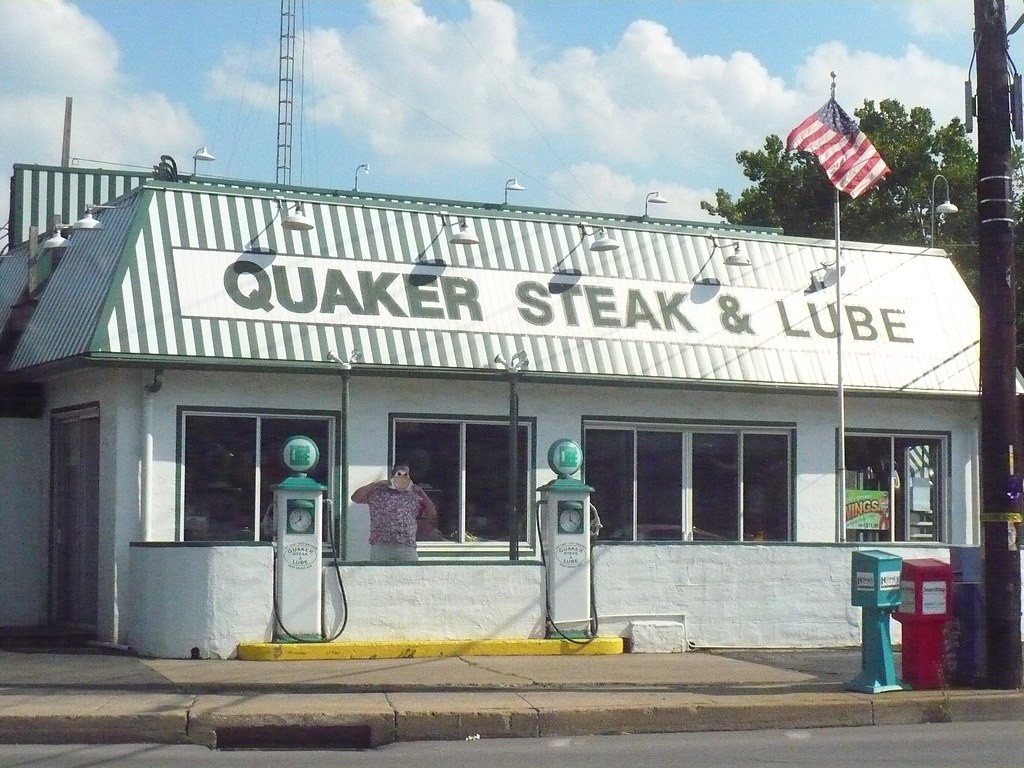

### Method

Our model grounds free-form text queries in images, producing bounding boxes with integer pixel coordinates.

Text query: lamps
[930,174,957,249]
[327,348,364,370]
[643,191,668,217]
[579,222,620,251]
[275,194,314,231]
[440,211,481,244]
[44,203,117,250]
[495,351,529,373]
[502,179,526,205]
[710,233,753,267]
[193,146,216,178]
[820,246,847,287]
[353,163,370,191]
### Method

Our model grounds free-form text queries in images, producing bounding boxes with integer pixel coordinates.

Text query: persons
[351,463,434,561]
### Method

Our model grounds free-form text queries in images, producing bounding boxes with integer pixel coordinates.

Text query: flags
[787,100,893,200]
